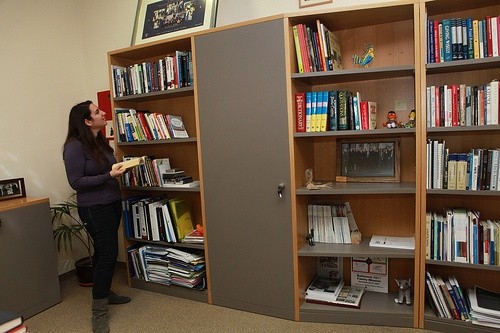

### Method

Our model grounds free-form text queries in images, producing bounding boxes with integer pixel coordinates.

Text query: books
[307,274,365,309]
[427,16,500,63]
[426,80,500,126]
[425,208,500,266]
[114,108,189,142]
[109,48,194,98]
[426,138,500,192]
[425,267,500,329]
[111,156,147,172]
[295,89,376,132]
[293,19,343,73]
[0,310,29,333]
[126,243,206,291]
[369,234,415,250]
[308,199,360,244]
[121,194,205,244]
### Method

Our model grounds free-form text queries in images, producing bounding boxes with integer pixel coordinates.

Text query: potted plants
[50,191,98,287]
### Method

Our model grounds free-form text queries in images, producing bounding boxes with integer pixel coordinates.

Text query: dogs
[394,277,412,304]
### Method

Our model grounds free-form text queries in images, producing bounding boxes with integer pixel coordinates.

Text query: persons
[63,100,132,333]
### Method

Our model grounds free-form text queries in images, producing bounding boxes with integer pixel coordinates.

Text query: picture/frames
[336,136,404,182]
[0,177,26,201]
[130,0,217,46]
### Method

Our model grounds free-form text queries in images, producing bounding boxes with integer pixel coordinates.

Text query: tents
[123,156,199,188]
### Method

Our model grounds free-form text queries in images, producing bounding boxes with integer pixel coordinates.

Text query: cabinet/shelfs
[103,0,500,333]
[0,196,63,320]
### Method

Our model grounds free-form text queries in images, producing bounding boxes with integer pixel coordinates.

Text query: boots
[91,298,110,333]
[109,290,130,304]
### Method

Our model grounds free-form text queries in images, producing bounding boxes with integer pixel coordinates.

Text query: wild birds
[352,41,376,68]
